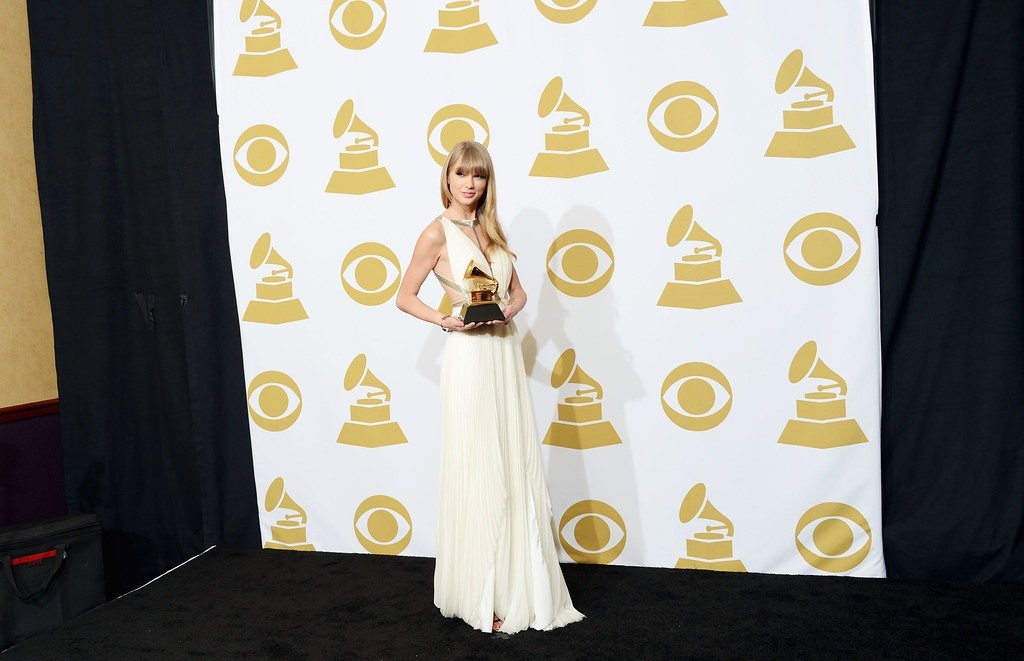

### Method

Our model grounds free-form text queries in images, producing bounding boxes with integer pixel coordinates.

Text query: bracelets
[441,315,454,332]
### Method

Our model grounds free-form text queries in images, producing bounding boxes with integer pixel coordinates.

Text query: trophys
[458,258,506,324]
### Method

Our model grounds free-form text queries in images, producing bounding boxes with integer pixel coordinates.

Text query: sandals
[492,613,504,633]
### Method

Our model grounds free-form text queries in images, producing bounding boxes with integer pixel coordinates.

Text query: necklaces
[450,218,479,226]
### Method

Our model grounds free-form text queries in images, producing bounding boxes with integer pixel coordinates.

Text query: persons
[396,141,561,633]
